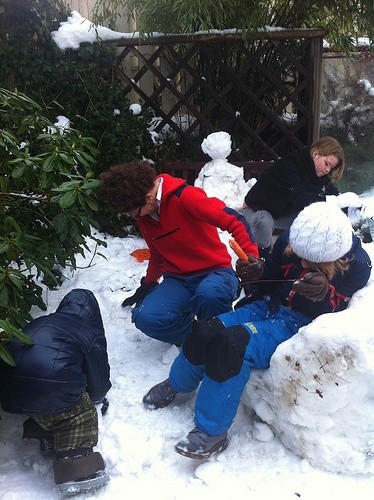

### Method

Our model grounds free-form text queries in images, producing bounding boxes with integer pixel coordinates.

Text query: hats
[289,202,352,263]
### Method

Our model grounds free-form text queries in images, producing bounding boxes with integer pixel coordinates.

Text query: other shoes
[258,246,274,276]
[40,436,54,456]
[52,472,109,498]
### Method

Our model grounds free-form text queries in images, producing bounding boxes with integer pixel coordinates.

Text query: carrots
[229,240,249,262]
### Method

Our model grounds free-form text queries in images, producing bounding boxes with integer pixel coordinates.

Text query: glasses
[124,200,144,220]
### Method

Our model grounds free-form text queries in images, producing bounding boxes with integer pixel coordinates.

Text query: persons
[95,161,261,410]
[143,202,372,461]
[0,288,111,497]
[246,137,345,258]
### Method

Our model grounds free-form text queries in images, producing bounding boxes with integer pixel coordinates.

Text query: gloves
[122,277,160,307]
[236,256,263,282]
[293,272,328,302]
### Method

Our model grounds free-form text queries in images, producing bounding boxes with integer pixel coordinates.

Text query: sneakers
[143,381,178,409]
[176,428,228,460]
